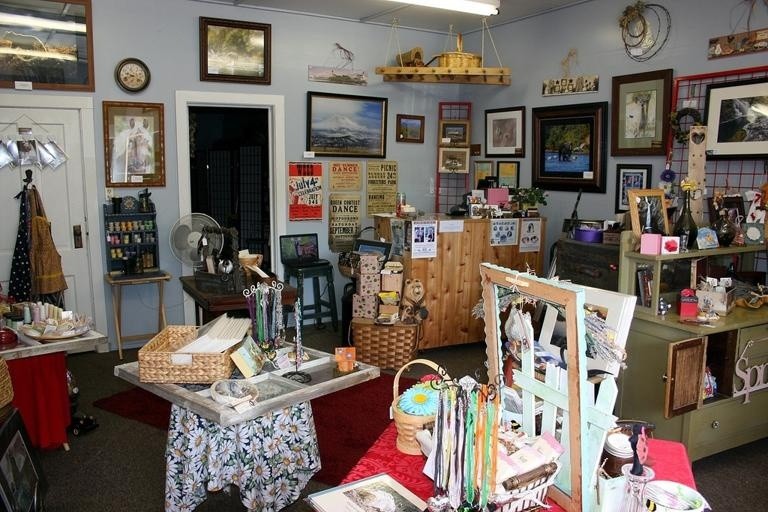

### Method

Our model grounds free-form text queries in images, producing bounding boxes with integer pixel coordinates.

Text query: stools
[283,259,339,332]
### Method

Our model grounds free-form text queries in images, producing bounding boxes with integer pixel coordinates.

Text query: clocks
[114,58,152,94]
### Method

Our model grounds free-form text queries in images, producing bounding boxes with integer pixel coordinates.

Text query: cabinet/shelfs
[103,196,161,274]
[618,230,768,462]
[554,239,691,297]
[374,211,547,349]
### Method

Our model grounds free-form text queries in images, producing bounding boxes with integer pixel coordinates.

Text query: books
[159,312,252,355]
[307,472,428,512]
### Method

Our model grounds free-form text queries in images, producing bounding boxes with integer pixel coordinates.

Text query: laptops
[278,233,329,269]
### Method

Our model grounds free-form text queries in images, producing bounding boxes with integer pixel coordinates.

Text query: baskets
[137,325,239,384]
[0,358,14,408]
[391,358,456,455]
[348,316,421,373]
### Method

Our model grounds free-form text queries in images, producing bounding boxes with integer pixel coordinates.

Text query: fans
[169,213,224,280]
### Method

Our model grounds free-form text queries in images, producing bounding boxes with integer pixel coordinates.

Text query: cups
[680,234,689,256]
[0,313,25,333]
[395,191,406,218]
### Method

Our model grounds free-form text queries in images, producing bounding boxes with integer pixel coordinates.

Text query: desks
[0,311,108,449]
[178,276,296,328]
[115,344,380,512]
[339,416,699,512]
[105,271,171,359]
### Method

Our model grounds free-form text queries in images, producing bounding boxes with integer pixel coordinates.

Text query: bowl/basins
[238,252,263,278]
[575,227,602,243]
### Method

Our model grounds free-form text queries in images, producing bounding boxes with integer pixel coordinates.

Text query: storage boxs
[677,295,699,320]
[602,232,621,245]
[356,255,380,296]
[381,262,404,292]
[378,292,400,318]
[696,285,736,316]
[352,294,378,319]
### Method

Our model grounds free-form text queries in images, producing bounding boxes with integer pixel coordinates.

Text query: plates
[641,481,706,512]
[20,311,93,340]
[604,432,633,460]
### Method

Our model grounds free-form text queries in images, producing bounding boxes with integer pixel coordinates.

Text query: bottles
[710,211,735,247]
[395,192,405,217]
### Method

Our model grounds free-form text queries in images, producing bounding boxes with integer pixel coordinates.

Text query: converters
[317,324,327,329]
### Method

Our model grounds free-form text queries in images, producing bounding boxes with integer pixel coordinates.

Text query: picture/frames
[0,408,41,511]
[439,120,471,148]
[103,101,166,187]
[473,160,494,190]
[199,15,272,85]
[484,105,526,159]
[496,161,520,189]
[637,271,653,307]
[531,101,609,194]
[0,0,95,93]
[708,196,746,226]
[610,68,674,156]
[396,114,425,144]
[615,164,652,214]
[438,148,471,174]
[703,75,768,160]
[306,91,388,158]
[629,188,669,235]
